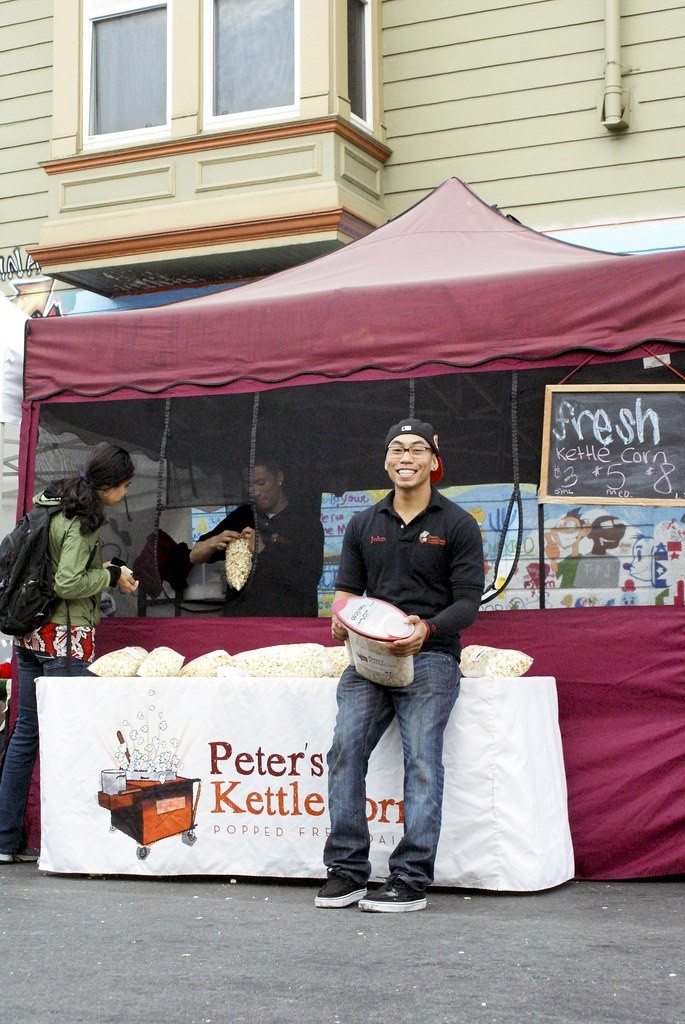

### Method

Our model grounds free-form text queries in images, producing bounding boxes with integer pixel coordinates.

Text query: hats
[384,418,443,486]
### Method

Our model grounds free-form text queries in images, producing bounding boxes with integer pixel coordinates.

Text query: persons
[189,451,325,618]
[0,438,140,860]
[314,419,483,912]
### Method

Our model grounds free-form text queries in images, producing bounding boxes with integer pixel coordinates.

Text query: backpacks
[1,501,100,637]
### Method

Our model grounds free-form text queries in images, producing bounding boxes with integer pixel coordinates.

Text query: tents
[5,175,685,881]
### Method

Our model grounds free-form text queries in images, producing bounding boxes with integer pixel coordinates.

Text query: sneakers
[1,845,39,862]
[315,870,367,908]
[358,875,427,913]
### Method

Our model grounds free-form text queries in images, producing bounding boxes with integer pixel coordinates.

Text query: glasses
[386,446,433,459]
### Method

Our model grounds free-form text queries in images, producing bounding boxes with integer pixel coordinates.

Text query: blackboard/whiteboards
[537,384,685,509]
